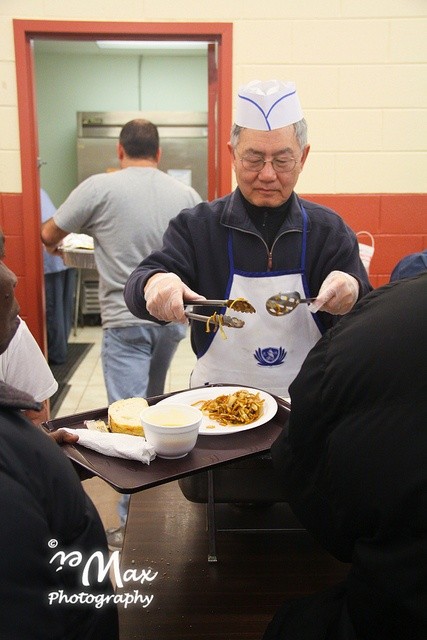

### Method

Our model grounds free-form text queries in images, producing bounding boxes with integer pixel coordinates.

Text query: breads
[107,397,149,436]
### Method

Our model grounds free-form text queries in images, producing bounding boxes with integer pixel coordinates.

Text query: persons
[41,119,204,552]
[0,314,59,427]
[40,186,76,365]
[288,271,427,639]
[123,79,374,406]
[0,230,121,638]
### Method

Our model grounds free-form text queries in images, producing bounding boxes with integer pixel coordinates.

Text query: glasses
[234,147,303,172]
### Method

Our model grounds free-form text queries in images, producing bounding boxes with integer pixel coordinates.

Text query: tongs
[183,297,256,330]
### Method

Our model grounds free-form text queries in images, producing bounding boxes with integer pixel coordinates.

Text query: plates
[158,386,278,437]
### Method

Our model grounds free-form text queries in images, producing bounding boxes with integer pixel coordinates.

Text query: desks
[115,479,353,638]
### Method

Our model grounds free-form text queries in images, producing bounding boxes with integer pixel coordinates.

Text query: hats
[234,80,304,131]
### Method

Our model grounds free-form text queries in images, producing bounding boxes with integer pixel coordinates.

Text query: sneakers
[105,527,125,553]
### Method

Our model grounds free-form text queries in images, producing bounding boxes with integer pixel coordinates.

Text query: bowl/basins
[139,404,203,460]
